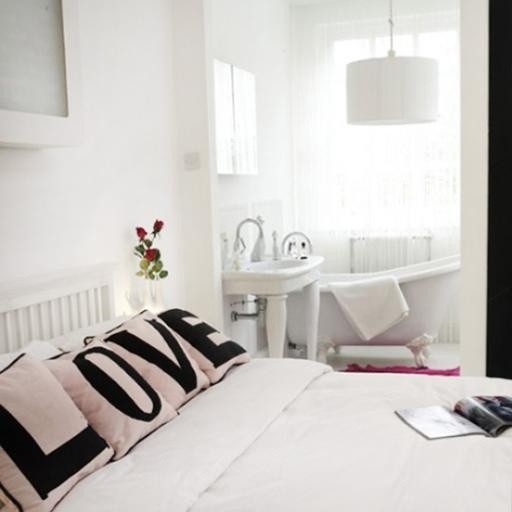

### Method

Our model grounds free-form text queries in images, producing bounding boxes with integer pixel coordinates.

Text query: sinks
[222,254,325,296]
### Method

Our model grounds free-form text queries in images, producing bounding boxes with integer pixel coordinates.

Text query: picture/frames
[0,0,83,149]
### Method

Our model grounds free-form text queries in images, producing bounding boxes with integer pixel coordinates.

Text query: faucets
[281,231,312,256]
[233,218,265,270]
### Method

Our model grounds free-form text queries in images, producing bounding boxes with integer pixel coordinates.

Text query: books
[392,394,512,442]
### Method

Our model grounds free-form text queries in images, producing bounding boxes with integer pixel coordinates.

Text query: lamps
[345,0,441,127]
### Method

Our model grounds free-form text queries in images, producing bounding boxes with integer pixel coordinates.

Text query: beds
[1,263,511,512]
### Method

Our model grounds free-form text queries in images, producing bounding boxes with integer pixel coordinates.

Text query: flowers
[132,220,169,305]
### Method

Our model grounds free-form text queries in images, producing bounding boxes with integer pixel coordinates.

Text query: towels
[325,275,410,343]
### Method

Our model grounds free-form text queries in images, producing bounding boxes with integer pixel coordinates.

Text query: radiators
[348,235,431,273]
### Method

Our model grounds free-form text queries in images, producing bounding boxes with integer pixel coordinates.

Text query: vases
[146,272,159,307]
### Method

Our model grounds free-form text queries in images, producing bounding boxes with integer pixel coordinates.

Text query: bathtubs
[285,254,462,347]
